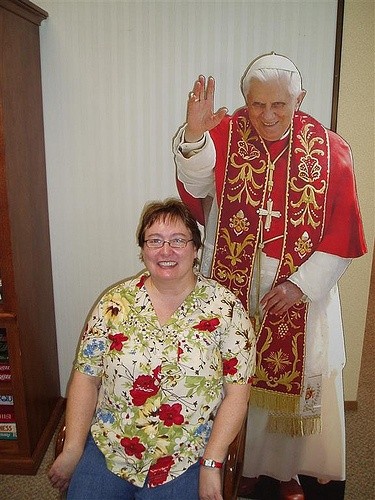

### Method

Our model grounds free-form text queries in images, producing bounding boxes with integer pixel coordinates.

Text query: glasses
[143,237,196,249]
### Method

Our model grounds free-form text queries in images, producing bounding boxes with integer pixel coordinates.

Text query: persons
[46,199,255,500]
[173,52,369,499]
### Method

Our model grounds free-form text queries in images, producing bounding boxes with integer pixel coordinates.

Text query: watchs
[199,459,224,469]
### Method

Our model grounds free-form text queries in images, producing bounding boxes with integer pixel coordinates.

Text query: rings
[191,92,199,103]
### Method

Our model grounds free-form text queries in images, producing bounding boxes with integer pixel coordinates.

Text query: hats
[240,51,303,82]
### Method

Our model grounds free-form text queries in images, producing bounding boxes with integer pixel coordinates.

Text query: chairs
[56,401,249,500]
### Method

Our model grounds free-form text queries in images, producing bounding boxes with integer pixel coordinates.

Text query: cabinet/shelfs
[0,0,68,476]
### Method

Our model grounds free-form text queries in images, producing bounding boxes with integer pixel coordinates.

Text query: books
[0,330,19,442]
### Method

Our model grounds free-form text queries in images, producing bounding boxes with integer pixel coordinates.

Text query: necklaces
[255,142,291,232]
